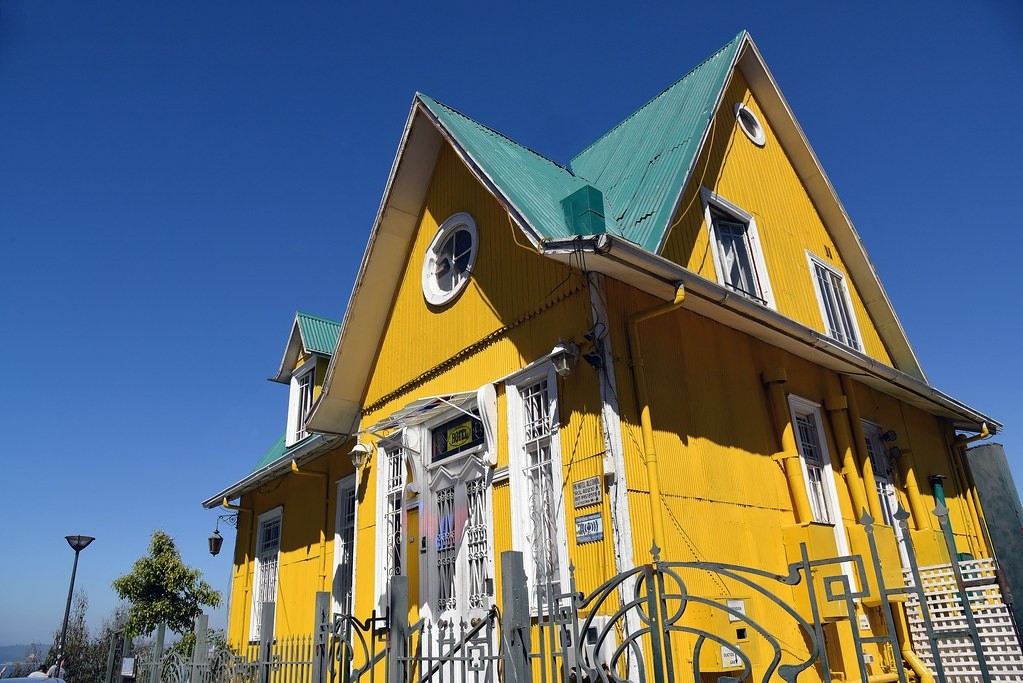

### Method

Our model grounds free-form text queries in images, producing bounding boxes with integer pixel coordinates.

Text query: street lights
[54,534,95,678]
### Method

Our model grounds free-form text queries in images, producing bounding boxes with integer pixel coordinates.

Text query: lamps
[347,434,374,470]
[208,514,239,556]
[881,429,897,443]
[545,336,580,379]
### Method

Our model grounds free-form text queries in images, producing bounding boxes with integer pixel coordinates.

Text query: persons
[27,665,49,679]
[46,655,68,680]
